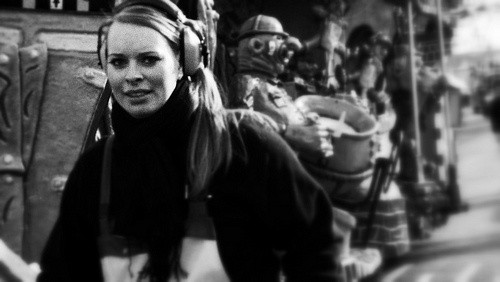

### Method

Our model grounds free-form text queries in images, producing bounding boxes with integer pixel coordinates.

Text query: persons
[483,94,500,148]
[232,12,471,259]
[32,0,348,282]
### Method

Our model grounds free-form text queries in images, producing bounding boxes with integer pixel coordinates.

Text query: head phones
[93,0,211,76]
